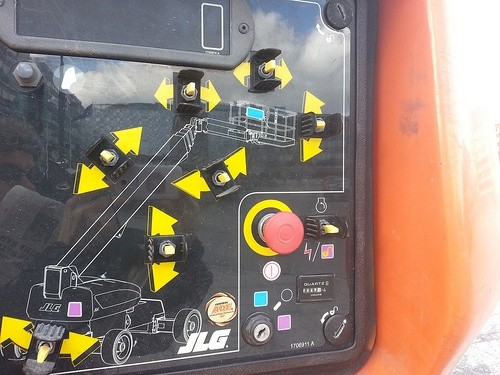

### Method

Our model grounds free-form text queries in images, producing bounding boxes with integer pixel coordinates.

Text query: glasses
[0,162,46,186]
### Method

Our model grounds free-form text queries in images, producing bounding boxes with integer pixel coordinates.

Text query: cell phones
[114,162,182,203]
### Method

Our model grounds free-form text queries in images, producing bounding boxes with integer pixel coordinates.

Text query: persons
[0,116,213,375]
